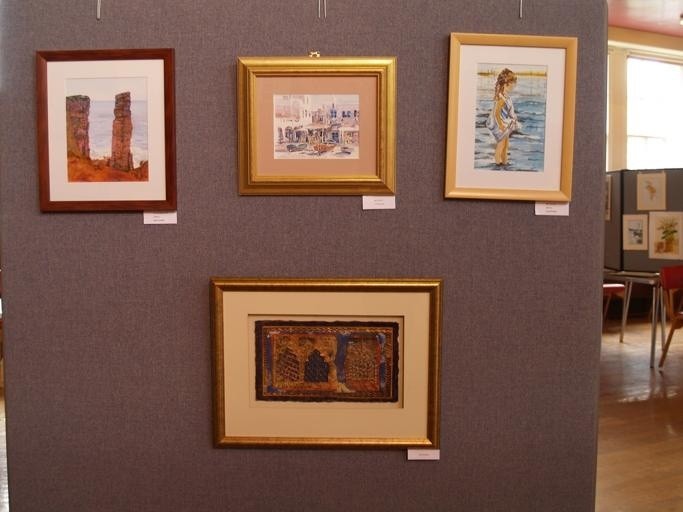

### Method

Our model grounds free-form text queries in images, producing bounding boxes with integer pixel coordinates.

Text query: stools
[602,281,626,336]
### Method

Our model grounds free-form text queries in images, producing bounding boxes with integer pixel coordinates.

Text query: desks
[608,269,669,368]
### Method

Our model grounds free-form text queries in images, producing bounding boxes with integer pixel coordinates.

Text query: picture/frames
[647,210,683,261]
[443,29,579,204]
[620,212,649,252]
[206,276,444,451]
[32,47,179,215]
[605,175,613,222]
[635,172,668,212]
[231,50,398,198]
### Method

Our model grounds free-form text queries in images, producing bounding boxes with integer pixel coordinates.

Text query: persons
[485,68,521,168]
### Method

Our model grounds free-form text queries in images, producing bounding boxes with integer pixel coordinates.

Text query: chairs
[657,265,683,369]
